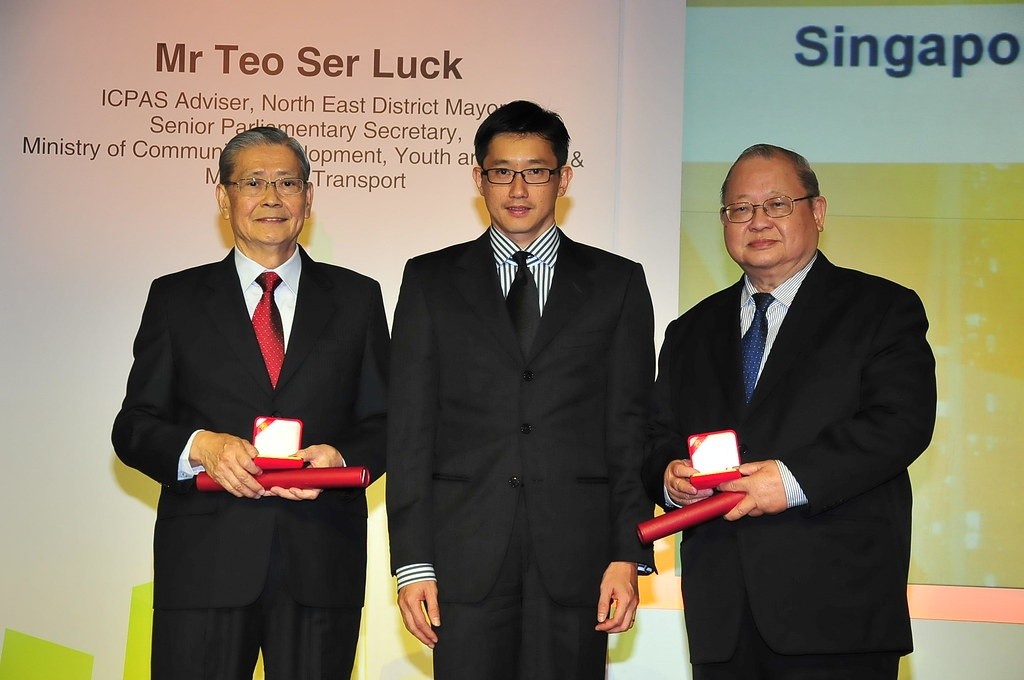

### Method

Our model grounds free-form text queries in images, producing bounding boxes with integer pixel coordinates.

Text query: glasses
[480,165,562,184]
[722,195,818,223]
[220,177,308,196]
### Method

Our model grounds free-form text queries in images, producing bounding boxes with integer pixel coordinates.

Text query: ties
[505,250,540,366]
[251,271,285,391]
[740,293,775,409]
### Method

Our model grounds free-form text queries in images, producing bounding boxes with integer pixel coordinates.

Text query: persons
[643,143,937,680]
[385,99,657,680]
[111,127,390,680]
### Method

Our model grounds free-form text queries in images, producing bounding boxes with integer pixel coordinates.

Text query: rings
[632,619,634,622]
